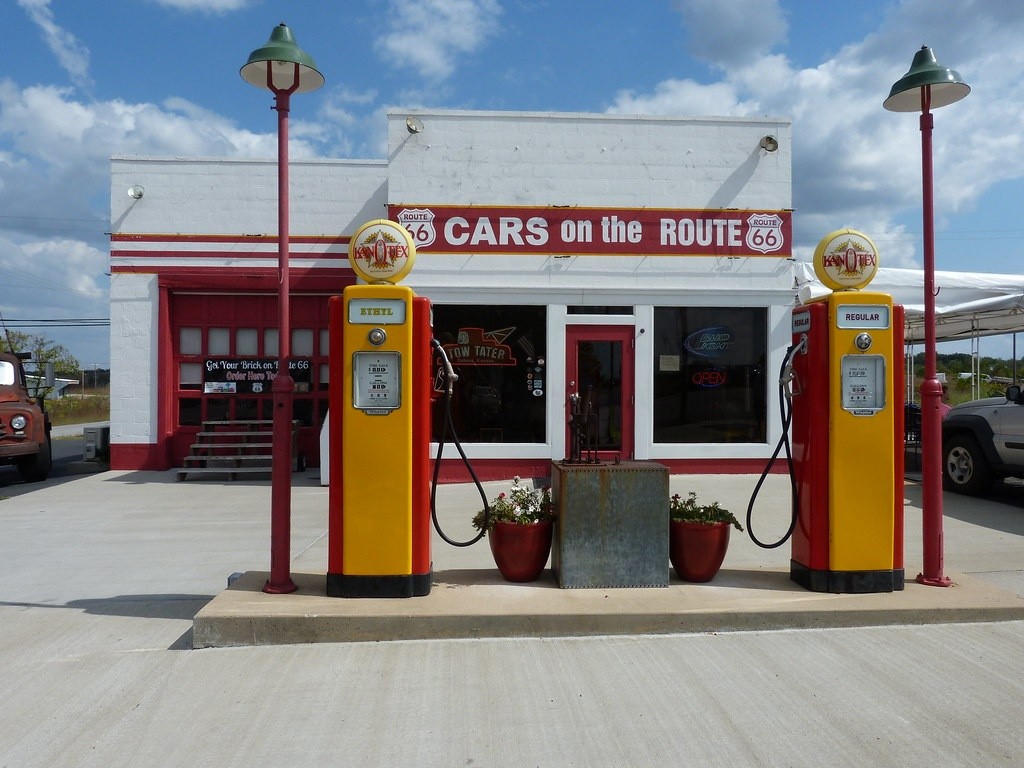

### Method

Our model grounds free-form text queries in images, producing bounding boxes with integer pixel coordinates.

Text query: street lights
[240,20,327,597]
[881,43,973,589]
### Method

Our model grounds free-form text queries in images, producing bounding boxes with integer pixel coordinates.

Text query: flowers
[669,490,744,532]
[472,475,552,531]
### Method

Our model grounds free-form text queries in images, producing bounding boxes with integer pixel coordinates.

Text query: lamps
[126,184,145,201]
[761,135,778,152]
[405,115,424,134]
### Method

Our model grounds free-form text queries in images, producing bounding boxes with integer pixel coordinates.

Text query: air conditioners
[84,427,111,462]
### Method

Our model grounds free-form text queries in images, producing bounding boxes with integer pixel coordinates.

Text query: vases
[669,520,730,583]
[489,513,553,583]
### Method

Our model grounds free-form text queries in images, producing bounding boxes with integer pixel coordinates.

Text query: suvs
[941,385,1024,497]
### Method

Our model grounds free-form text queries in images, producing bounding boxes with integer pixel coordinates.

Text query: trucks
[0,352,55,482]
[958,373,993,384]
